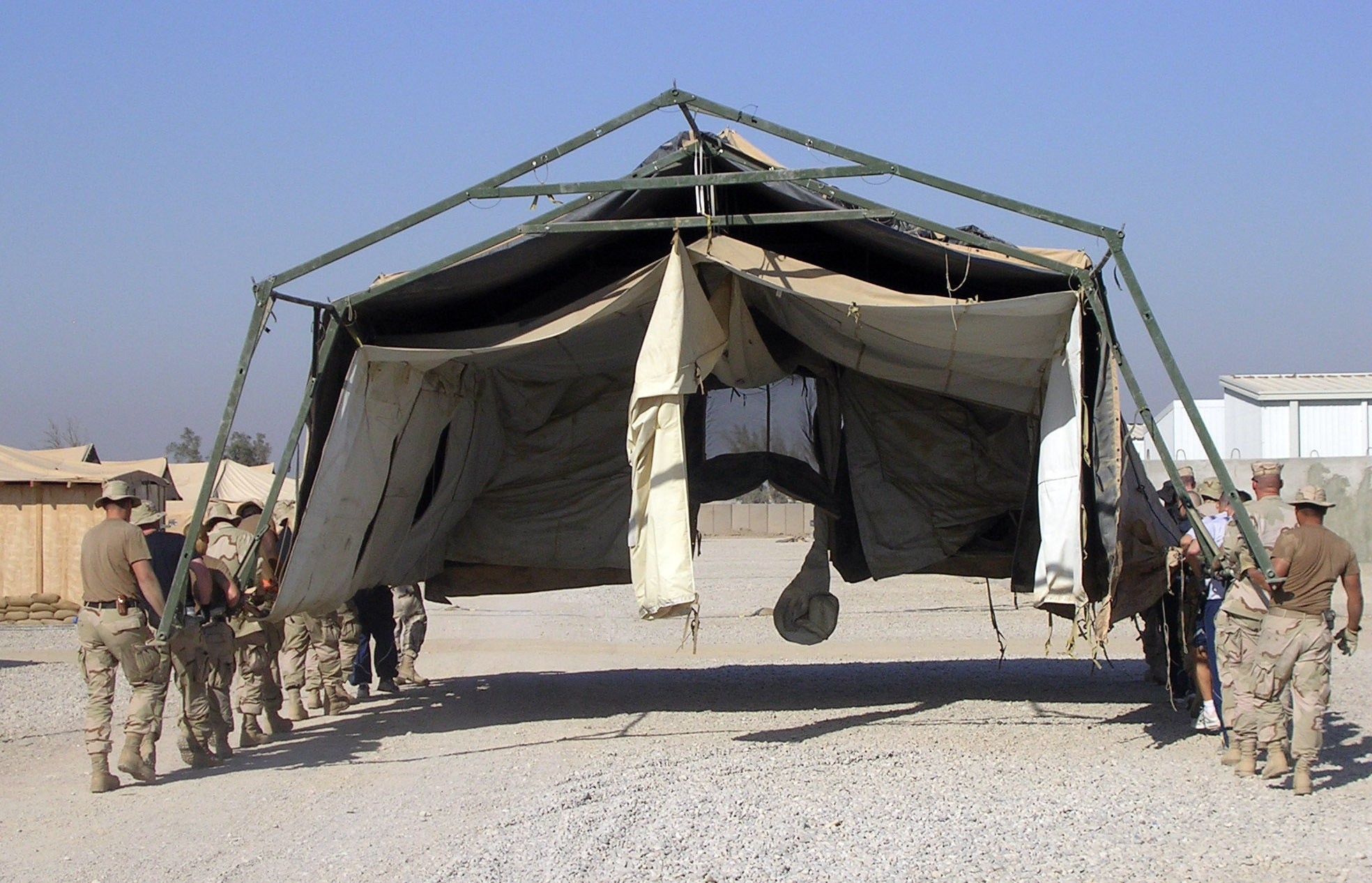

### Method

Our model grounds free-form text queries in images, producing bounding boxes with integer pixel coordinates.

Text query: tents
[212,90,1188,751]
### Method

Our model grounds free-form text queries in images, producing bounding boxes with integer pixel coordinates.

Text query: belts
[200,614,227,624]
[1269,606,1327,620]
[82,597,143,611]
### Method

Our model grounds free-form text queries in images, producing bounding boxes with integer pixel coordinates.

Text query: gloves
[1332,626,1359,658]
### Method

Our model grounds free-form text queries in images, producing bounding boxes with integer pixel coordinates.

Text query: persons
[1214,458,1300,767]
[1235,483,1365,798]
[1126,455,1256,747]
[181,521,237,757]
[73,475,176,793]
[122,501,224,771]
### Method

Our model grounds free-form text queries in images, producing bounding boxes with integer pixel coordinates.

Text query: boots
[88,753,122,793]
[242,716,273,748]
[1280,730,1290,760]
[1263,746,1287,779]
[1295,760,1313,796]
[399,656,429,686]
[137,733,155,773]
[1218,730,1234,756]
[284,689,310,722]
[308,688,322,709]
[1221,737,1243,766]
[193,737,222,767]
[116,731,158,782]
[393,675,406,686]
[1234,743,1255,779]
[264,709,294,733]
[333,683,360,704]
[212,730,235,760]
[177,719,201,768]
[324,684,350,716]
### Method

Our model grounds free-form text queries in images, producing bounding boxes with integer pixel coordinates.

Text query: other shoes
[1142,664,1201,718]
[375,678,400,693]
[1191,701,1221,733]
[357,682,372,700]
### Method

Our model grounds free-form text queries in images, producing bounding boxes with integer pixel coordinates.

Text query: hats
[1251,459,1282,476]
[272,499,295,525]
[1172,466,1196,478]
[237,498,265,518]
[1197,478,1228,500]
[1289,487,1336,509]
[1155,481,1177,502]
[94,481,140,508]
[204,503,240,530]
[127,501,167,526]
